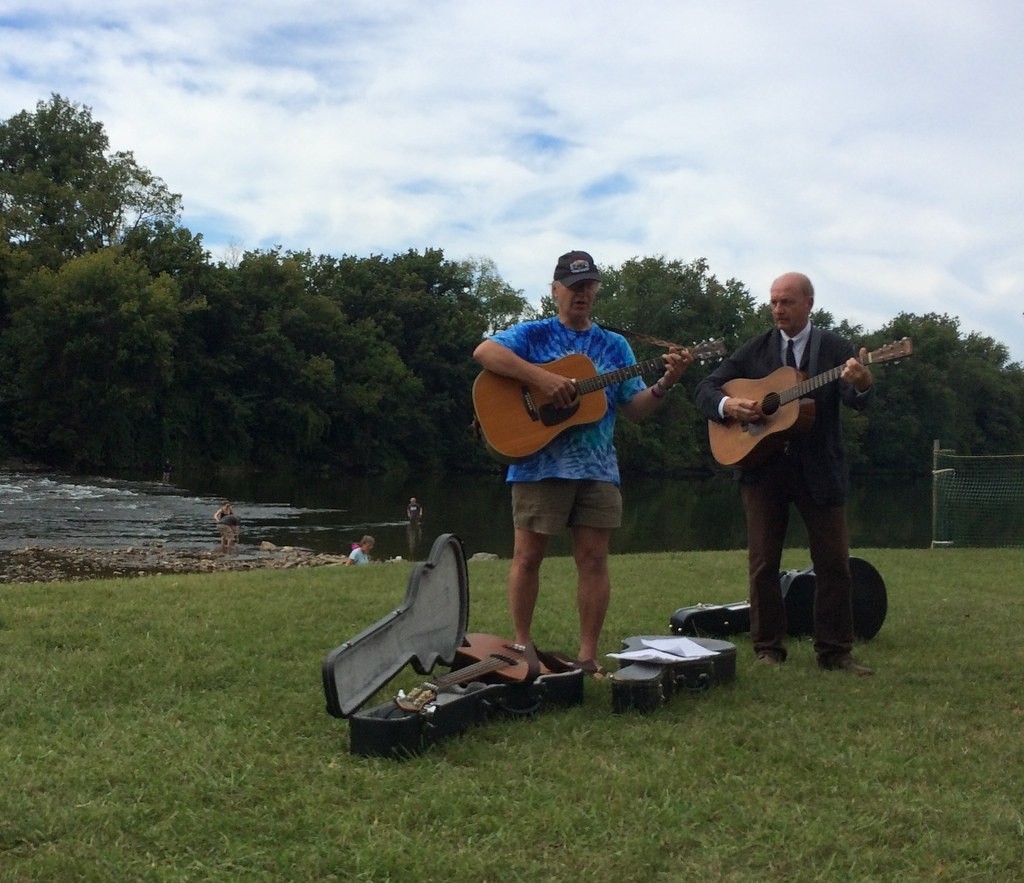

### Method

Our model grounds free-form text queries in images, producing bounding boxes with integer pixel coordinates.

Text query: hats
[553,250,602,288]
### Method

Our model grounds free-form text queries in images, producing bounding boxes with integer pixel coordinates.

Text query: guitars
[709,335,913,464]
[392,632,549,713]
[471,336,727,458]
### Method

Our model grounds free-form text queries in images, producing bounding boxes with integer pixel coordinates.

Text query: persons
[407,498,423,531]
[214,504,233,521]
[346,535,375,566]
[216,515,242,556]
[162,460,172,483]
[472,250,693,681]
[691,271,877,677]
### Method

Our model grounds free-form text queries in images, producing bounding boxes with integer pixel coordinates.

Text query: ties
[786,339,797,369]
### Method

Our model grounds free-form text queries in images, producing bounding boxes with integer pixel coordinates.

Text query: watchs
[657,377,676,392]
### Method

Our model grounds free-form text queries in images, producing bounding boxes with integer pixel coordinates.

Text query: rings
[672,364,678,368]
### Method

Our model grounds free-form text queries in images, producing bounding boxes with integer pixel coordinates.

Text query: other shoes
[819,660,875,677]
[590,666,612,681]
[760,653,779,664]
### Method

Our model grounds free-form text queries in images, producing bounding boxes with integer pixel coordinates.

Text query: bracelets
[651,386,666,399]
